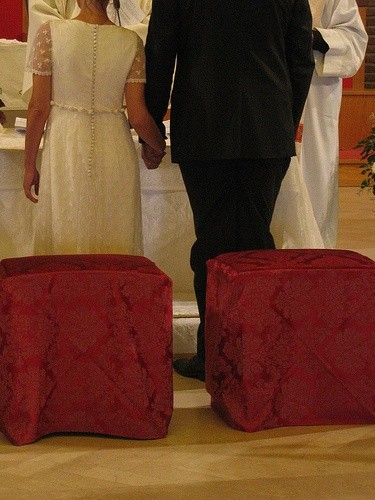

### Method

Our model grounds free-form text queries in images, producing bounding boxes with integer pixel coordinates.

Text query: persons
[143,0,315,383]
[23,0,166,256]
[25,1,152,132]
[286,0,369,249]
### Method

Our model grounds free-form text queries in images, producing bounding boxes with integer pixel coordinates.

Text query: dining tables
[0,39,28,108]
[0,127,284,299]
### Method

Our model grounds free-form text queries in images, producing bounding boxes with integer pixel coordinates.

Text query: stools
[206,249,375,433]
[0,253,173,446]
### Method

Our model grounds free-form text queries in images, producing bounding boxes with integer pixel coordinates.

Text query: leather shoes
[174,358,205,383]
